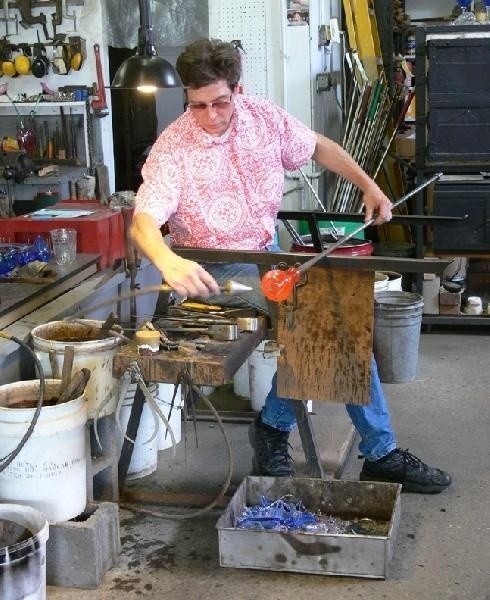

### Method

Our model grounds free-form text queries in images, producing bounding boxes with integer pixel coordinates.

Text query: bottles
[17,122,36,155]
[467,296,482,315]
[407,32,416,55]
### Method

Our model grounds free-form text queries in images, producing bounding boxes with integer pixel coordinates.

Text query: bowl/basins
[208,324,239,341]
[236,315,260,332]
[135,330,161,356]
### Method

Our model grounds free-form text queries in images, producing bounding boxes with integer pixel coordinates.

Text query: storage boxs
[0,199,126,270]
[215,474,403,579]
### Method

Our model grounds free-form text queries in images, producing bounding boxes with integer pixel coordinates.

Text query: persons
[127,37,452,494]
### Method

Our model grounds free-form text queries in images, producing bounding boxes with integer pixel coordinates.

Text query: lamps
[110,0,185,96]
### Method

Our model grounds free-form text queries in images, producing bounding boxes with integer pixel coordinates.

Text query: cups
[50,227,78,266]
[37,192,59,210]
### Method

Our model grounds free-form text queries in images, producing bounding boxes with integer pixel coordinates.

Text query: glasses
[187,93,233,110]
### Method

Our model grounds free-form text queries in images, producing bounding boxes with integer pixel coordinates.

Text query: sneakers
[359,447,452,492]
[248,411,295,476]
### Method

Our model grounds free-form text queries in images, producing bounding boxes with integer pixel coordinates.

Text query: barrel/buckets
[155,380,182,450]
[0,378,89,527]
[374,241,417,290]
[0,503,51,599]
[289,233,374,257]
[194,339,312,413]
[374,290,424,382]
[90,384,159,481]
[374,272,389,291]
[375,269,403,292]
[31,316,125,418]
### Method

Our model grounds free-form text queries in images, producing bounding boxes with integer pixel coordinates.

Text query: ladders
[342,0,414,244]
